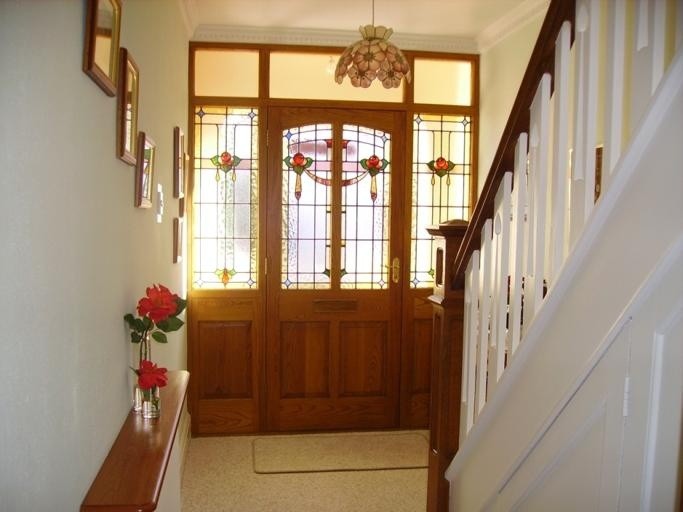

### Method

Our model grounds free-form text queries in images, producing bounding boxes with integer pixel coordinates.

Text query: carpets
[252,432,430,474]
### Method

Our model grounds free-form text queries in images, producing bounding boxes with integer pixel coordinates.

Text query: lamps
[335,0,412,88]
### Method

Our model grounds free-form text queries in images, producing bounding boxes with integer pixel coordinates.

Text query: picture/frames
[83,0,156,208]
[174,127,185,263]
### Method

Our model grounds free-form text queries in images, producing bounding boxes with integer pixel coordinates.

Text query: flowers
[129,359,169,410]
[124,284,188,368]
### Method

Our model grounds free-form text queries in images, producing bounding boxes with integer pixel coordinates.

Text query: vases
[141,386,160,418]
[134,384,161,412]
